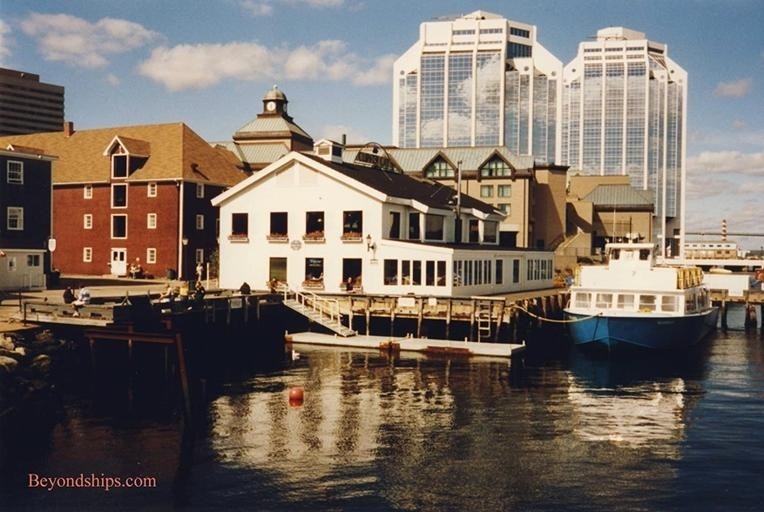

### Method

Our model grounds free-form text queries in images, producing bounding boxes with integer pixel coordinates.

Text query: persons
[195,261,205,282]
[69,283,90,317]
[158,283,173,303]
[176,279,190,302]
[133,264,142,279]
[62,283,77,304]
[190,281,206,299]
[236,281,251,295]
[268,277,278,295]
[127,263,136,278]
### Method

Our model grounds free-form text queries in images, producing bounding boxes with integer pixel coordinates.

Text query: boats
[564,243,721,365]
